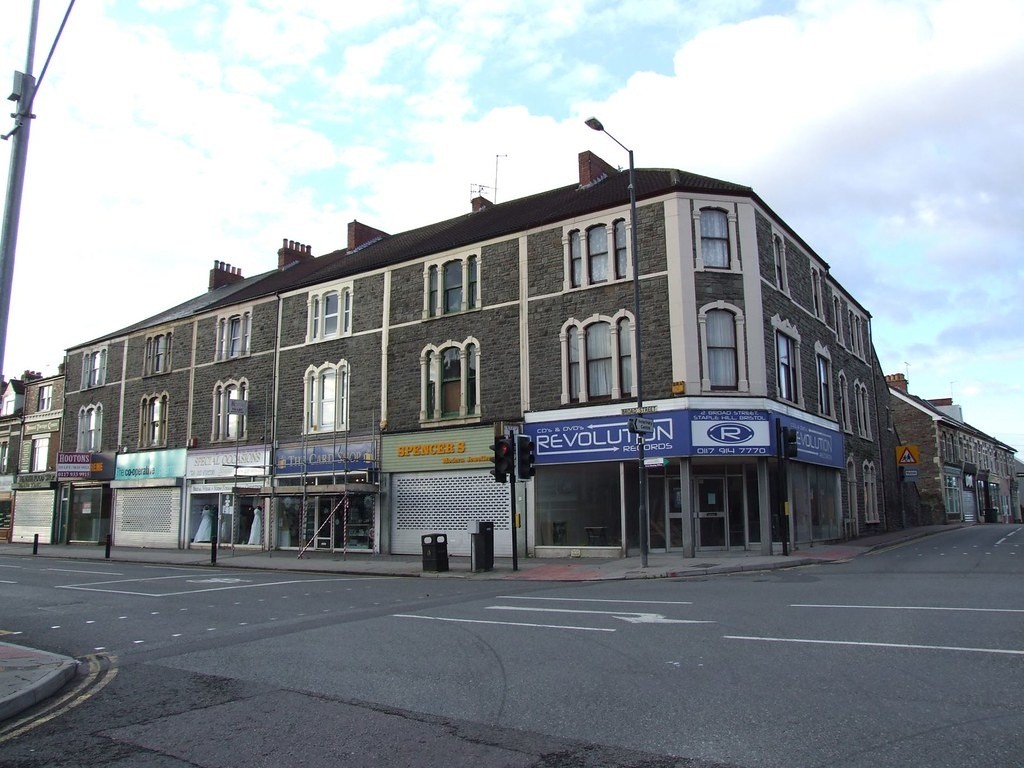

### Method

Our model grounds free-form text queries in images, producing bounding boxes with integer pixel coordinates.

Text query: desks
[583,527,608,546]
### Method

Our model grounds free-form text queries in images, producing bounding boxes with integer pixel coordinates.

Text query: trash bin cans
[467,521,494,573]
[990,509,998,522]
[421,532,449,572]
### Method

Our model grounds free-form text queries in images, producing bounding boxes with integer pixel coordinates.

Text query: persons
[194,503,217,542]
[241,505,262,545]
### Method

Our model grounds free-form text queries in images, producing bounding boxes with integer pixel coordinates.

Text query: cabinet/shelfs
[346,524,372,549]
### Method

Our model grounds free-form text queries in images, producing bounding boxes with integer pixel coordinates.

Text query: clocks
[494,421,524,451]
